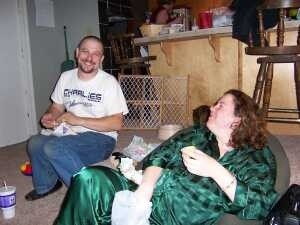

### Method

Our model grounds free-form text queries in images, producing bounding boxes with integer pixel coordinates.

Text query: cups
[199,13,212,28]
[0,186,16,219]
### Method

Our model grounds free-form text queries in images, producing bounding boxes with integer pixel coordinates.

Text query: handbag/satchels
[266,184,300,225]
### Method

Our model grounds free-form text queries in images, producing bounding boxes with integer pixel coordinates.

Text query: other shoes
[25,182,61,202]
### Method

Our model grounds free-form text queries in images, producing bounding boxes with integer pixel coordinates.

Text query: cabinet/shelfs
[98,0,148,80]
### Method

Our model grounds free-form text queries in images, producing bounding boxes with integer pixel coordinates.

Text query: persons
[24,36,130,202]
[52,88,280,225]
[141,0,184,75]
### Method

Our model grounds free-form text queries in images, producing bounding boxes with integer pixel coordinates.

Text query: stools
[108,32,157,125]
[245,0,300,118]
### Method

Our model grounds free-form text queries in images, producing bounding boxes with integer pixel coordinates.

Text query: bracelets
[222,175,236,192]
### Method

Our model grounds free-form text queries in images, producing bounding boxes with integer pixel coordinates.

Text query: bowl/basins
[158,124,183,141]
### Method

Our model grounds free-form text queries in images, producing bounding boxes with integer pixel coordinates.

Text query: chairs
[215,130,291,225]
